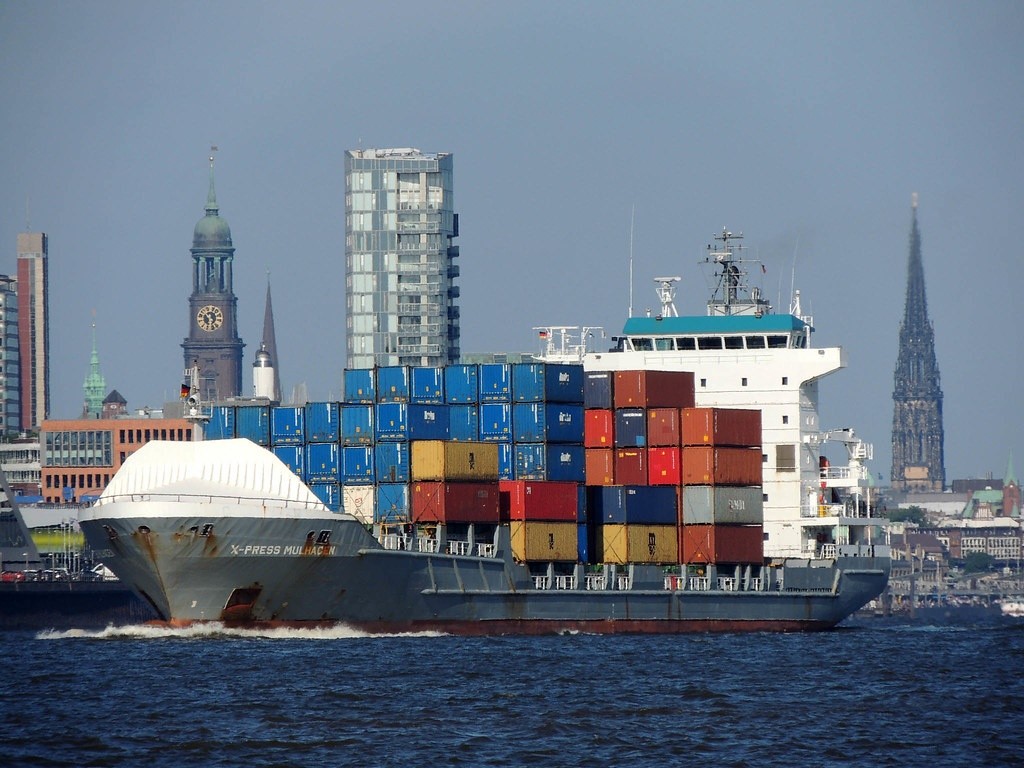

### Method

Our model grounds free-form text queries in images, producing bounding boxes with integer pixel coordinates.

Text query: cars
[0,570,24,583]
[72,569,100,581]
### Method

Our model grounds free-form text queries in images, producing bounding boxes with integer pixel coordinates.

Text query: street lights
[23,553,28,570]
[49,554,55,582]
[75,554,81,581]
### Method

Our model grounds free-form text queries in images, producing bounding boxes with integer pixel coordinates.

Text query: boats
[77,436,894,640]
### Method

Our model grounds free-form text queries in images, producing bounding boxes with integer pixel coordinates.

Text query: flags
[179,384,191,399]
[538,331,550,341]
[588,332,595,339]
[761,264,766,273]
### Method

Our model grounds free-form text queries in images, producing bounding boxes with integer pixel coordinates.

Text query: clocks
[197,305,224,331]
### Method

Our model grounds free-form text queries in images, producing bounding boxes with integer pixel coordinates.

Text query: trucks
[89,563,119,581]
